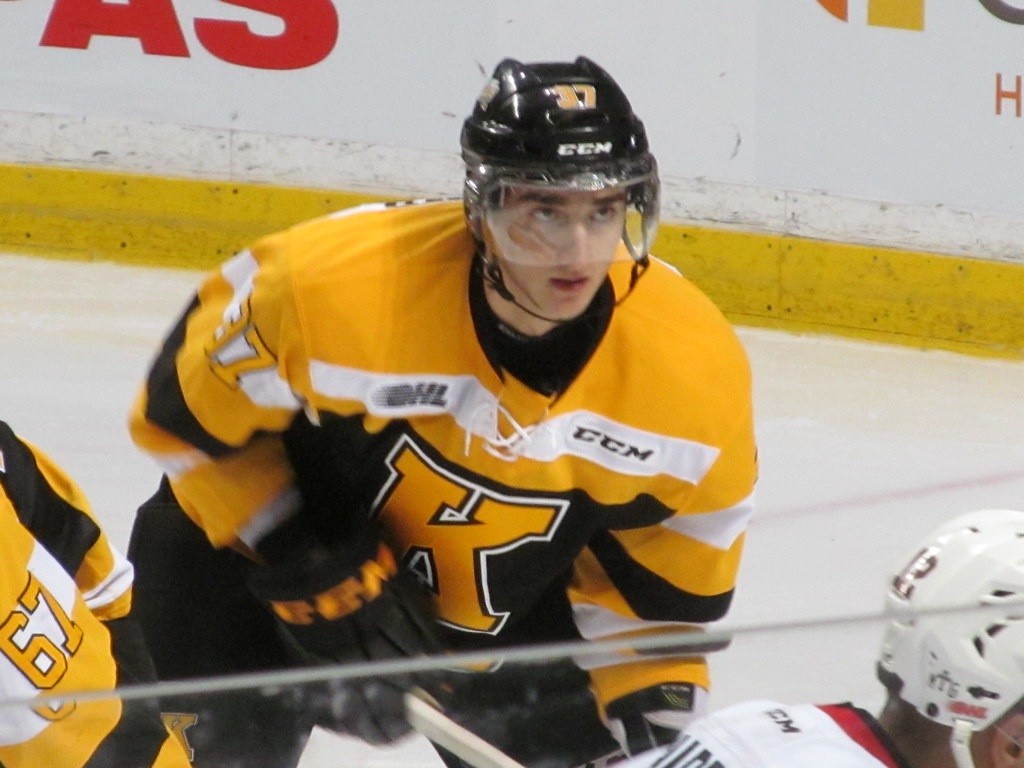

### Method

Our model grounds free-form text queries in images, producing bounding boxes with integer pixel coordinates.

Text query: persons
[0,420,192,768]
[127,55,758,768]
[651,508,1024,768]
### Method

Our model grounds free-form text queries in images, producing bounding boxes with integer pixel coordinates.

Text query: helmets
[879,511,1024,729]
[460,57,657,192]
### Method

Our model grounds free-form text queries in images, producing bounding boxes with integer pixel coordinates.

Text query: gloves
[250,497,451,741]
